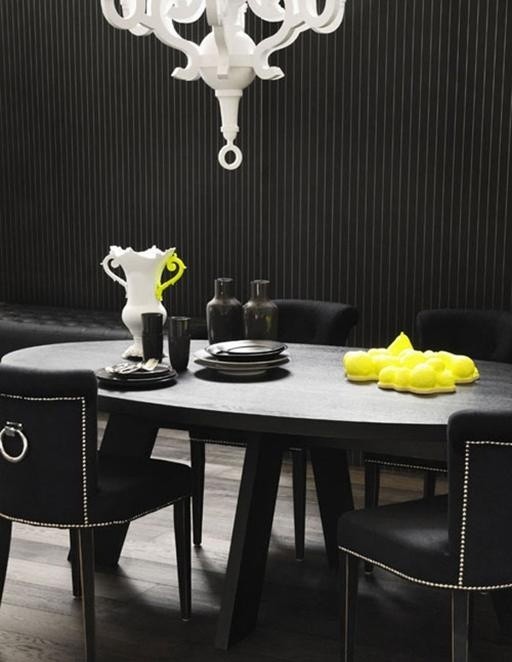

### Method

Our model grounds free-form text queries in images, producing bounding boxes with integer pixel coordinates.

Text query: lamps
[101,0,346,173]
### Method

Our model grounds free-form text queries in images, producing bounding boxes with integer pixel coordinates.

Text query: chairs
[338,408,512,662]
[189,295,359,561]
[361,303,512,587]
[0,363,192,662]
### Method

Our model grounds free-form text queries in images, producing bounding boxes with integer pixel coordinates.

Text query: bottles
[243,278,280,342]
[206,276,242,344]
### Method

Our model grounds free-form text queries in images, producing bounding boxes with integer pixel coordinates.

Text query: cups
[141,312,164,364]
[167,316,191,371]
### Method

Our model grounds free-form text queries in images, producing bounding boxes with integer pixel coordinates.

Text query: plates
[95,365,180,391]
[193,339,290,376]
